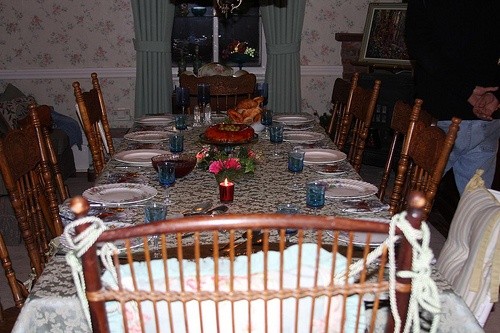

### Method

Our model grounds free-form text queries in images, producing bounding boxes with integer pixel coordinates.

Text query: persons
[403,0,500,226]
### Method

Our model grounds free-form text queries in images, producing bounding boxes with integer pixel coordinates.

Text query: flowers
[222,39,259,63]
[195,145,262,179]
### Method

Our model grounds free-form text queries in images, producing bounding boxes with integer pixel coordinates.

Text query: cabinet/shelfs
[334,31,364,81]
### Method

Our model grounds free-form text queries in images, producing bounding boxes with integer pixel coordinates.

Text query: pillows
[0,94,39,131]
[435,168,500,326]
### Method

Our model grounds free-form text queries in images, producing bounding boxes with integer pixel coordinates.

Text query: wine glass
[144,204,166,259]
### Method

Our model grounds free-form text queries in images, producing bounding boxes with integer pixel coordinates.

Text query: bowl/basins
[151,153,197,178]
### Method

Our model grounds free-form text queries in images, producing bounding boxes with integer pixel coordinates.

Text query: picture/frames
[357,2,414,69]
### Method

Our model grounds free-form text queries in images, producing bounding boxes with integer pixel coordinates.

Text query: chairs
[389,97,461,225]
[13,102,75,236]
[0,233,28,333]
[203,81,253,112]
[378,101,440,203]
[68,191,429,333]
[0,98,64,274]
[326,77,352,141]
[71,72,116,178]
[338,71,381,172]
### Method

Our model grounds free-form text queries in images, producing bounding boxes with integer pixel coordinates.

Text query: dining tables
[9,114,487,333]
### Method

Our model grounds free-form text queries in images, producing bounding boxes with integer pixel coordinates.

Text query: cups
[219,182,234,203]
[175,115,187,130]
[277,204,299,236]
[306,180,326,209]
[169,134,184,153]
[157,163,176,188]
[270,126,283,143]
[261,110,272,125]
[288,150,305,173]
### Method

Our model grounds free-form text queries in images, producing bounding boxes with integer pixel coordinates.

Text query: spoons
[209,230,260,252]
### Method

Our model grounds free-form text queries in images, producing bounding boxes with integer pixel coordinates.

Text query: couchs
[176,63,259,114]
[0,78,83,196]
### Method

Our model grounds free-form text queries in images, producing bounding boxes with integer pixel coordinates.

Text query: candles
[219,179,235,204]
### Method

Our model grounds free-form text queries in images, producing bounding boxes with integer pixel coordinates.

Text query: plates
[136,116,175,125]
[326,217,401,246]
[271,113,314,126]
[112,149,171,166]
[82,182,158,204]
[59,221,152,252]
[319,178,378,199]
[212,111,227,119]
[124,131,174,143]
[283,130,325,143]
[300,149,347,165]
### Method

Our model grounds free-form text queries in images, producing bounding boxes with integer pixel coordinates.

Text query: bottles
[204,104,211,125]
[192,106,200,125]
